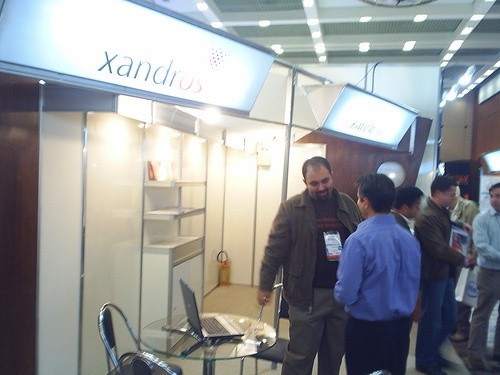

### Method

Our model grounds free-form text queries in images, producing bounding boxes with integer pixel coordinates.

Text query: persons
[257,156,363,375]
[333,174,421,375]
[388,176,500,375]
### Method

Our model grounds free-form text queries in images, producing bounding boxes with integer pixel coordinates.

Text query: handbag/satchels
[454,264,479,310]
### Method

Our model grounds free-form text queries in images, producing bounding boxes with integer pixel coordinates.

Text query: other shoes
[416,363,447,375]
[438,354,448,368]
[449,333,468,341]
[469,351,489,371]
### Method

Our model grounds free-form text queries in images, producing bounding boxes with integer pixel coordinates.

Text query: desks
[139,311,278,375]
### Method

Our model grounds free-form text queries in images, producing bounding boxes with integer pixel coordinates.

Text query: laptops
[179,279,244,339]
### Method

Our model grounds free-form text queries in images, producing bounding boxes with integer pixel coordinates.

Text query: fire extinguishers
[217,249,231,287]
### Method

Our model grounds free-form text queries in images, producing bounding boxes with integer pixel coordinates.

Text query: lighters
[245,339,262,346]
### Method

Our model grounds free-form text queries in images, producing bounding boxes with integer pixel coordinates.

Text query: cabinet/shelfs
[138,122,209,359]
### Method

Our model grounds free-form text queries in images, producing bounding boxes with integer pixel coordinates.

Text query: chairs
[239,283,289,375]
[98,301,183,375]
